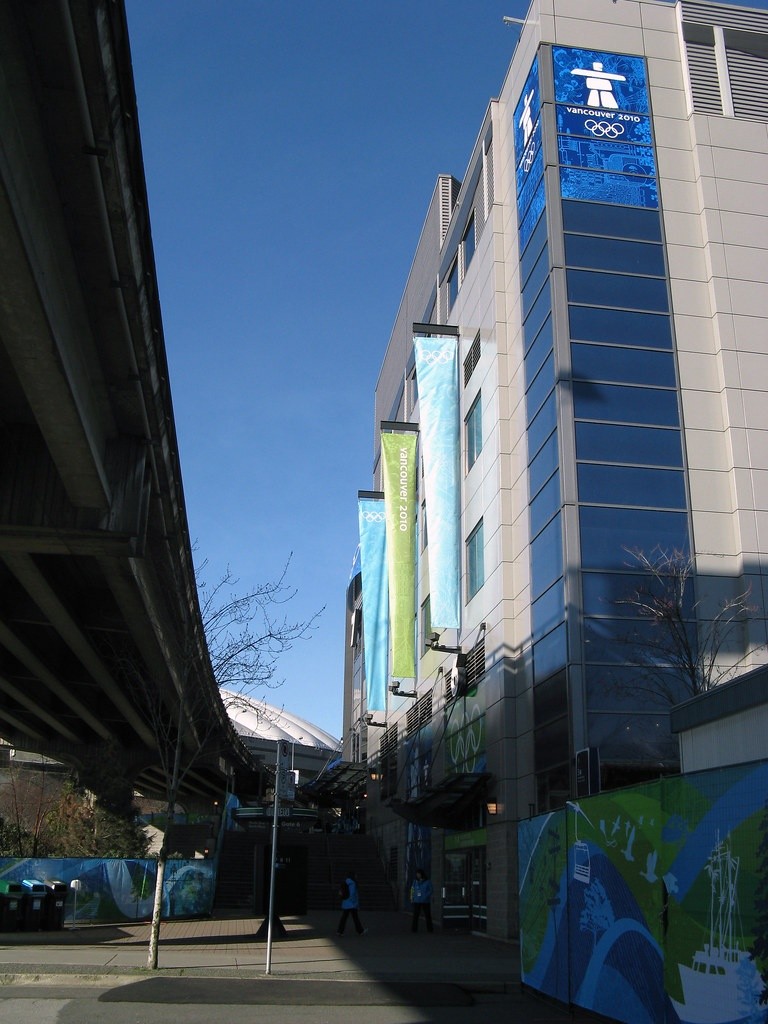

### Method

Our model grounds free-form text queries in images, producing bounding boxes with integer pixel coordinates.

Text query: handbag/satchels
[409,880,416,904]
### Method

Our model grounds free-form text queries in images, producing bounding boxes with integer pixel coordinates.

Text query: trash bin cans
[22,880,47,932]
[40,880,67,932]
[0,880,24,933]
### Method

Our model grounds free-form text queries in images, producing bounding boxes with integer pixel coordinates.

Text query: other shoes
[359,928,368,935]
[336,929,345,936]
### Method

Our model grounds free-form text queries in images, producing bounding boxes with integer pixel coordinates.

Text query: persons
[336,871,368,936]
[410,869,433,933]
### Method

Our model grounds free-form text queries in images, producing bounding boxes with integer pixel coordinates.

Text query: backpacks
[339,882,355,900]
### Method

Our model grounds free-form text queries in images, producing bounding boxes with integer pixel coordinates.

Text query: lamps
[362,714,387,727]
[424,633,462,654]
[388,679,417,698]
